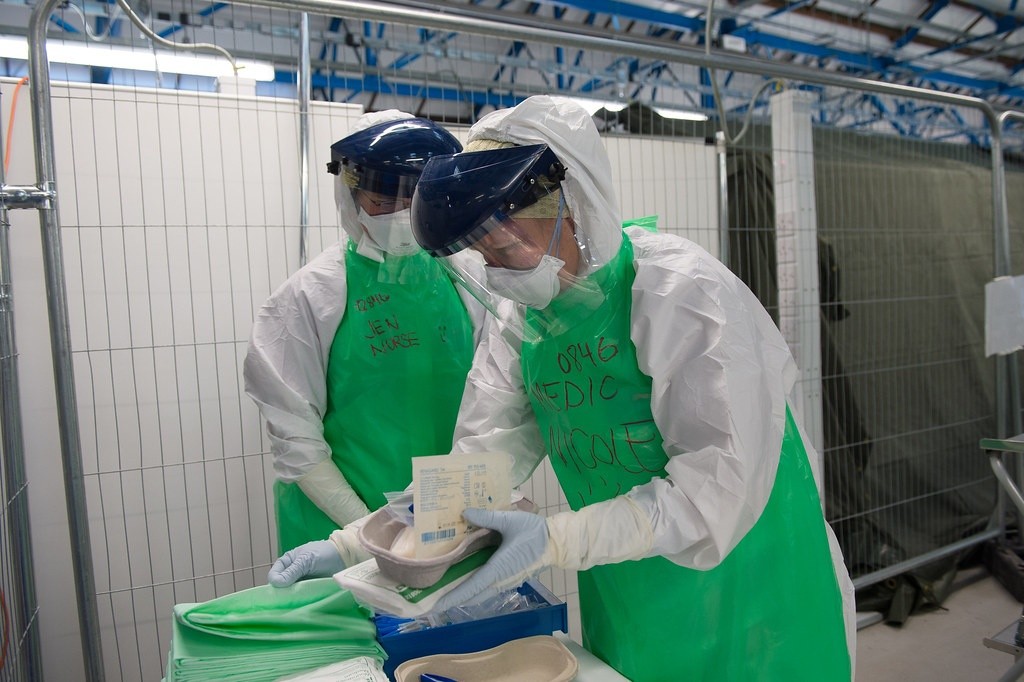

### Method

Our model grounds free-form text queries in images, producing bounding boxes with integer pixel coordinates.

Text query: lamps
[0,33,275,82]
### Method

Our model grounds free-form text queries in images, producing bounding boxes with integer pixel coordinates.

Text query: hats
[459,138,569,218]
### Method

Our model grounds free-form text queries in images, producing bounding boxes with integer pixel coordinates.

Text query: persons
[268,95,857,682]
[245,108,493,555]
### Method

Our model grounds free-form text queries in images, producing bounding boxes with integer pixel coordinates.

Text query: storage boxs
[373,568,568,680]
[357,490,539,589]
[394,635,580,682]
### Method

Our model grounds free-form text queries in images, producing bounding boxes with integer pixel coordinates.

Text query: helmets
[410,143,565,258]
[321,115,464,198]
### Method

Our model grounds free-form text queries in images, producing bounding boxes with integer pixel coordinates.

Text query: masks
[355,207,418,256]
[483,190,565,310]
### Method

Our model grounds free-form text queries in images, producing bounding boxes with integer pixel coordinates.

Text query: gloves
[267,540,346,587]
[436,507,556,625]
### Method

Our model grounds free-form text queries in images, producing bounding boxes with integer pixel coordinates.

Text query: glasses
[358,188,411,213]
